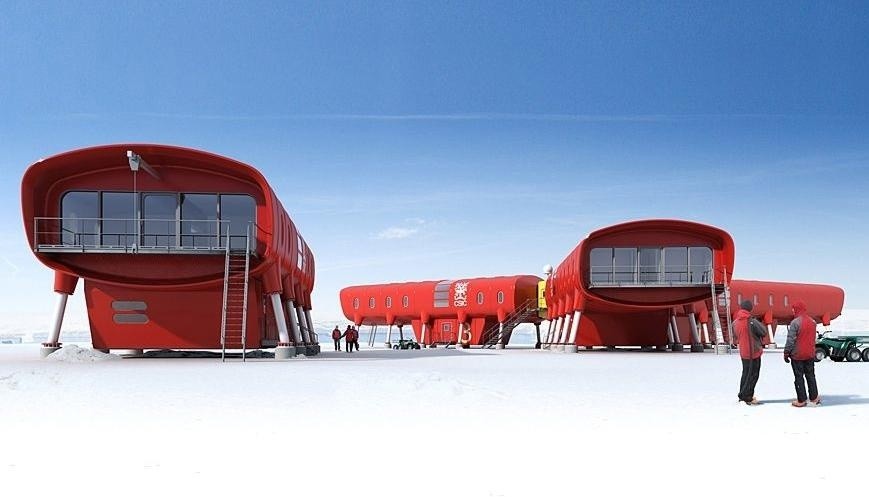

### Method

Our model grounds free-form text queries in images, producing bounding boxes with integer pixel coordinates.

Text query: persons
[784,302,820,406]
[332,325,341,351]
[732,300,767,404]
[338,324,355,352]
[352,324,359,351]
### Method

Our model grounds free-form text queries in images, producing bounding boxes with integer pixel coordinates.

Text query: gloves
[784,351,791,363]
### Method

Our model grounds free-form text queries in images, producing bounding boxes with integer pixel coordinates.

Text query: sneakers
[810,396,822,405]
[746,399,758,404]
[792,401,807,407]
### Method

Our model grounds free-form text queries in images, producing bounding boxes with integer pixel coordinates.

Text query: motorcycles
[814,330,862,361]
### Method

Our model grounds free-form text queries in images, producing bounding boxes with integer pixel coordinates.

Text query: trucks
[389,338,421,351]
